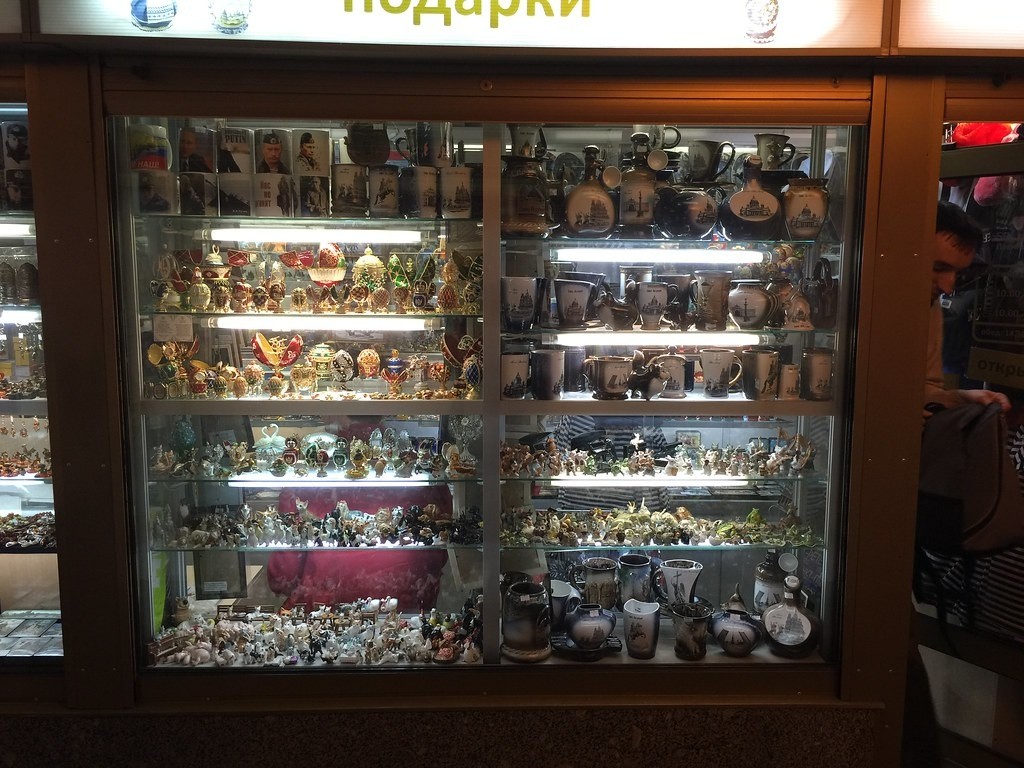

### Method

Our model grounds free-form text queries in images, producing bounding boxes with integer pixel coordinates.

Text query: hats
[6,169,32,188]
[7,124,28,139]
[263,134,283,144]
[301,133,315,144]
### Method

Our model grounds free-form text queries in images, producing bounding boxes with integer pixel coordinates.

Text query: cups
[685,138,736,182]
[632,125,681,152]
[0,120,36,213]
[126,118,474,221]
[498,553,717,663]
[753,131,796,170]
[777,551,799,573]
[647,149,669,171]
[501,338,834,401]
[505,121,546,158]
[598,165,622,190]
[501,260,733,333]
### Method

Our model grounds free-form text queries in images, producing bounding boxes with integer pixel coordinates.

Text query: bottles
[766,276,796,328]
[729,279,779,331]
[763,576,822,658]
[559,145,616,240]
[717,155,782,242]
[500,583,553,661]
[500,154,550,239]
[709,606,765,657]
[564,602,618,648]
[753,546,790,616]
[617,133,659,228]
[781,176,831,243]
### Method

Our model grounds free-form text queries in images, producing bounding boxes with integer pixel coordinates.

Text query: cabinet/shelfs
[103,109,858,680]
[0,102,67,660]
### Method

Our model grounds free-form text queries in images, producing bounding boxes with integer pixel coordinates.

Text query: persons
[139,132,329,217]
[778,412,831,592]
[555,413,672,577]
[268,416,454,616]
[6,124,33,211]
[897,200,1024,768]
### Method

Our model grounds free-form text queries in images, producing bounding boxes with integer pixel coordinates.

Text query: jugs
[652,183,725,241]
[798,258,838,329]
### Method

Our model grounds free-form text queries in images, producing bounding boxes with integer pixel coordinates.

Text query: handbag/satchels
[915,400,1024,560]
